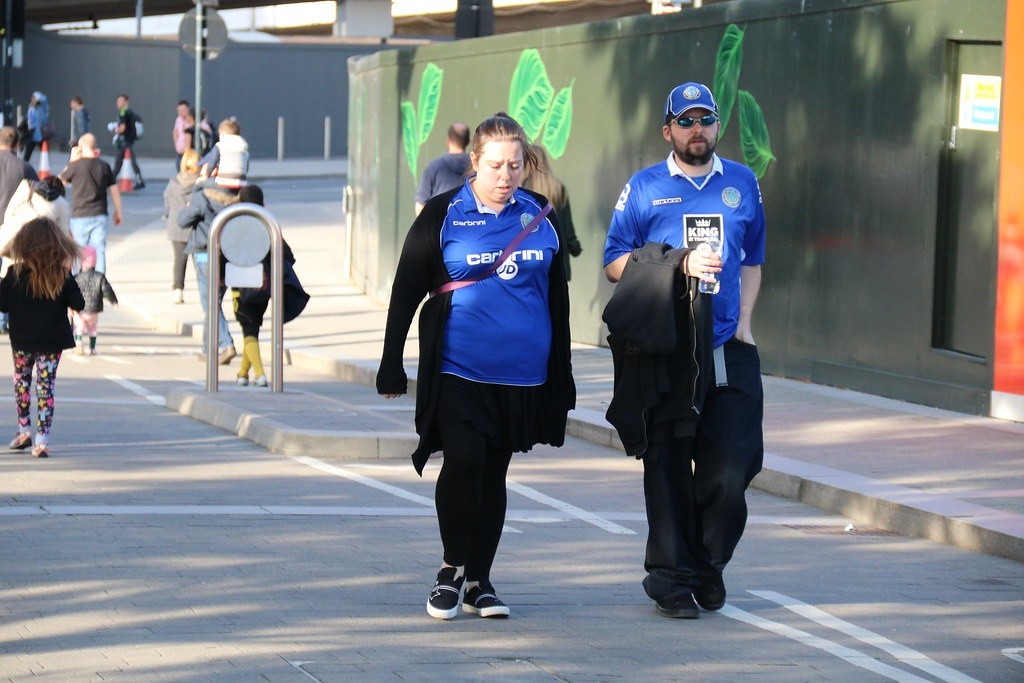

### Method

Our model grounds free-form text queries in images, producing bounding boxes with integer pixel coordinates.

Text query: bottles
[699,231,723,295]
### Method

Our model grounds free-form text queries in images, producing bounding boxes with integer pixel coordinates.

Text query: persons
[415,123,472,217]
[376,112,577,618]
[0,216,85,457]
[0,91,271,388]
[603,81,765,617]
[522,144,582,281]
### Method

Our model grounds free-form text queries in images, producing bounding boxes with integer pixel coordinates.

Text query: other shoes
[9,431,32,449]
[171,288,183,302]
[655,593,701,618]
[692,575,726,610]
[219,346,236,365]
[75,346,84,355]
[253,375,268,387]
[88,348,96,355]
[236,374,249,385]
[31,441,49,458]
[197,354,207,361]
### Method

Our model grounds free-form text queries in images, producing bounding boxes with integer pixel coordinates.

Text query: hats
[79,246,96,268]
[664,82,719,121]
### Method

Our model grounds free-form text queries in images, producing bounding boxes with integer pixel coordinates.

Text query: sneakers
[426,567,467,618]
[461,587,510,617]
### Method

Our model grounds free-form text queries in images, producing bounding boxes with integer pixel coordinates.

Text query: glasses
[667,115,720,128]
